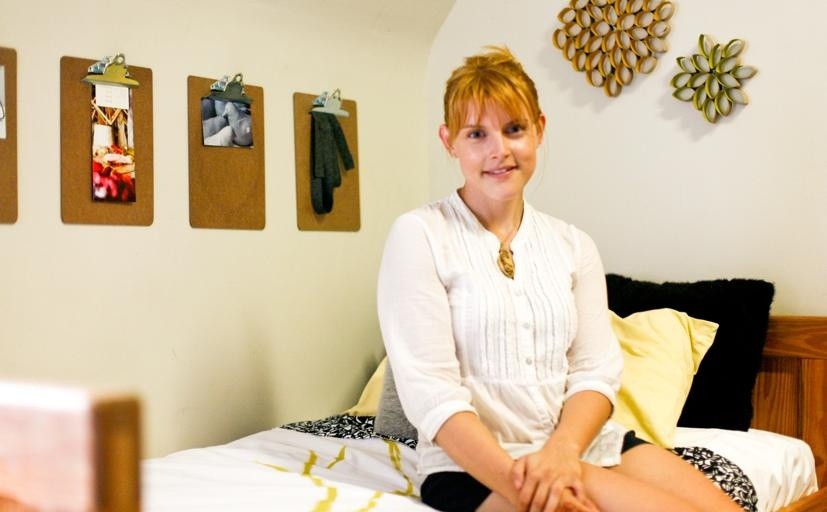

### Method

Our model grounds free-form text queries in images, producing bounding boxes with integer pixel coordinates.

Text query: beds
[0,312,826,511]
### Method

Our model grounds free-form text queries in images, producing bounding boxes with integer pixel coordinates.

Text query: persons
[372,41,742,512]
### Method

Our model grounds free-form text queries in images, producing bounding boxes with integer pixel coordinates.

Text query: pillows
[605,271,776,433]
[342,306,720,448]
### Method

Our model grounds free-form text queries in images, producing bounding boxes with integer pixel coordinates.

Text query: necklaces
[459,187,523,278]
